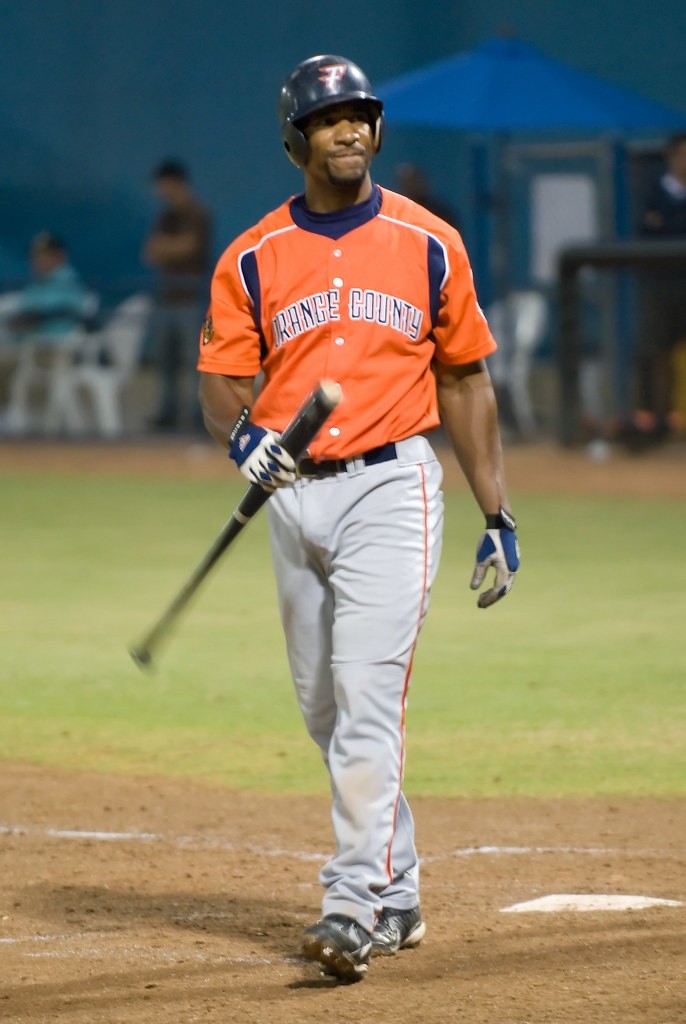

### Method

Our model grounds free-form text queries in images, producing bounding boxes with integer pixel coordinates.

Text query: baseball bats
[127,377,344,671]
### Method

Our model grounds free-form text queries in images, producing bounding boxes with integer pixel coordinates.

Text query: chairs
[6,291,151,439]
[484,289,548,438]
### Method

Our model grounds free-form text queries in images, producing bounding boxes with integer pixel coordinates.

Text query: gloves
[228,406,308,491]
[469,506,521,609]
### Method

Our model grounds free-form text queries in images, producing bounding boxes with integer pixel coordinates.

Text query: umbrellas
[370,36,686,274]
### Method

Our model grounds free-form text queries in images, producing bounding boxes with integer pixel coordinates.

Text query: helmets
[279,55,383,168]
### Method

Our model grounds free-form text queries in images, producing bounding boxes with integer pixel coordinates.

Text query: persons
[397,163,459,230]
[145,159,215,435]
[0,232,100,402]
[197,53,519,978]
[635,132,686,439]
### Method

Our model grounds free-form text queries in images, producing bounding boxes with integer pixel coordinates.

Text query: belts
[300,442,398,476]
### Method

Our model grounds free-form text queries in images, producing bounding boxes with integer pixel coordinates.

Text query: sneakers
[369,906,424,955]
[303,912,372,983]
